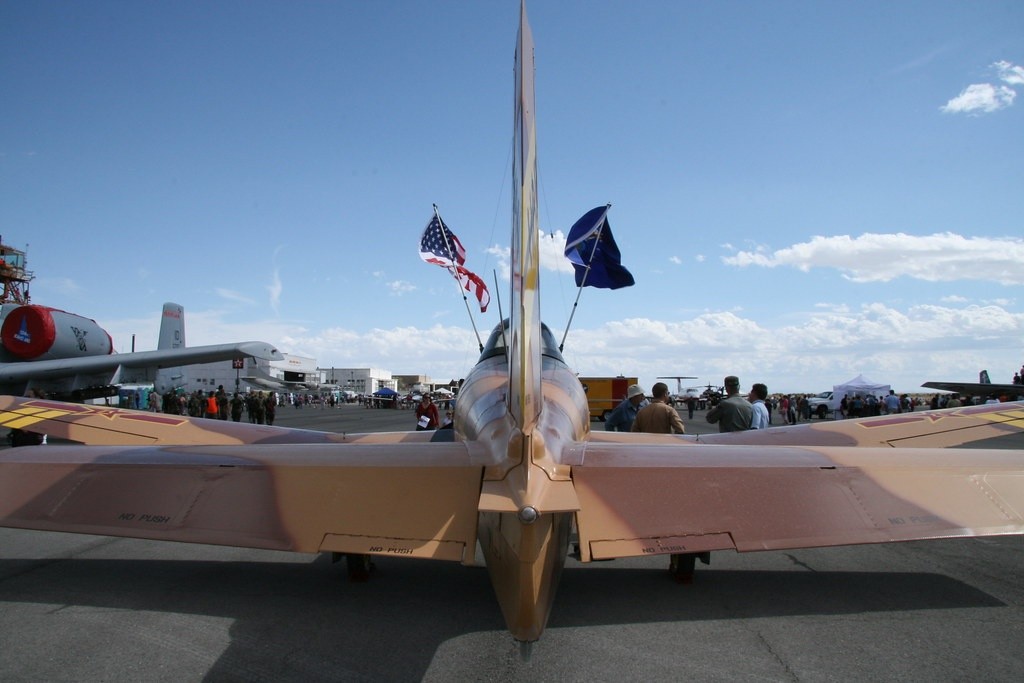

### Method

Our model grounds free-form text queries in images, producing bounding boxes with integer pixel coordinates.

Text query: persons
[687,396,695,419]
[293,392,340,409]
[437,393,456,426]
[148,383,228,421]
[416,392,439,431]
[706,376,753,433]
[1013,365,1024,384]
[357,392,420,410]
[765,393,811,425]
[840,389,915,419]
[230,389,294,426]
[604,384,645,433]
[748,383,770,430]
[929,392,1010,410]
[631,382,685,434]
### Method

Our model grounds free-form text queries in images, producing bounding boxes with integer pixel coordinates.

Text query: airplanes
[654,375,752,411]
[920,370,1024,406]
[0,300,285,407]
[0,0,1023,661]
[237,357,322,400]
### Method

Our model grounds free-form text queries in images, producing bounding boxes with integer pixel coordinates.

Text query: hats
[627,384,645,398]
[725,376,739,386]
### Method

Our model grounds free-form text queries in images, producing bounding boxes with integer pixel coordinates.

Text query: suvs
[803,391,835,419]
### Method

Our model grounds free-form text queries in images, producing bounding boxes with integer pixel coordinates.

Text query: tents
[373,387,401,397]
[430,388,455,396]
[832,373,891,419]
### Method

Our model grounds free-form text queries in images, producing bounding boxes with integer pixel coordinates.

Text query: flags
[417,212,491,313]
[564,205,636,289]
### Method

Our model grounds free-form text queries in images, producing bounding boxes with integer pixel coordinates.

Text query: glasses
[446,413,451,415]
[424,397,430,399]
